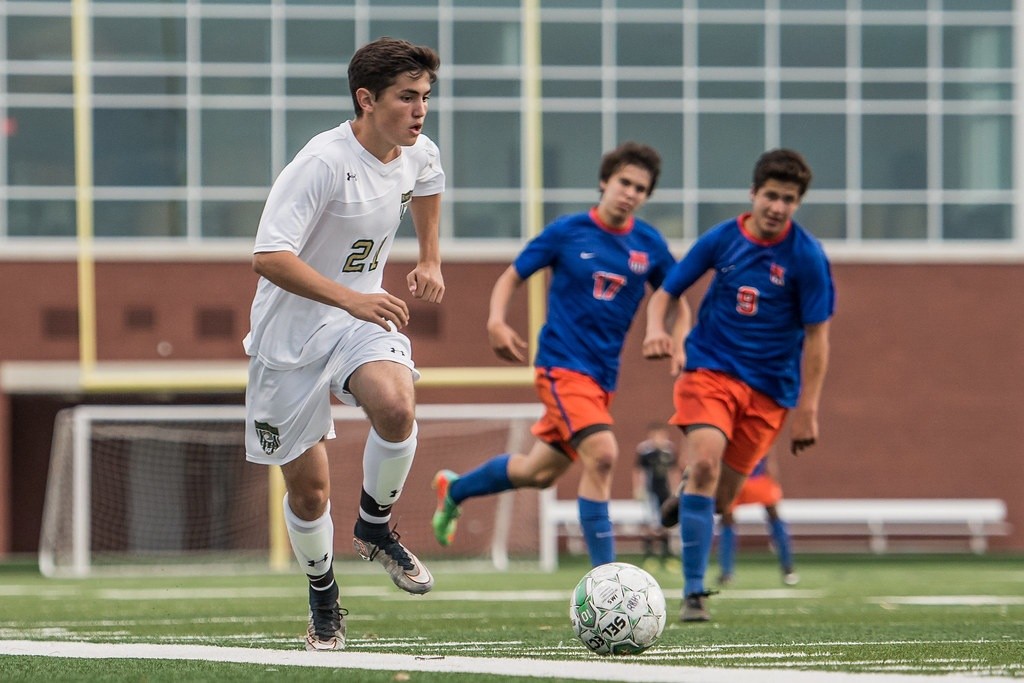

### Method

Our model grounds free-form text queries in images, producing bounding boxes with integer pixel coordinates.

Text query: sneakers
[659,463,694,528]
[352,516,435,597]
[716,573,732,584]
[782,573,799,585]
[430,469,461,549]
[305,593,349,651]
[679,595,710,622]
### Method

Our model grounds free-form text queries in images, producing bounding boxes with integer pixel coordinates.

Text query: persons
[631,419,685,570]
[642,147,836,627]
[431,140,688,567]
[241,37,449,648]
[713,442,800,587]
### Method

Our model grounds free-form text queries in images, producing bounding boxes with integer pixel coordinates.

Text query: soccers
[569,562,666,657]
